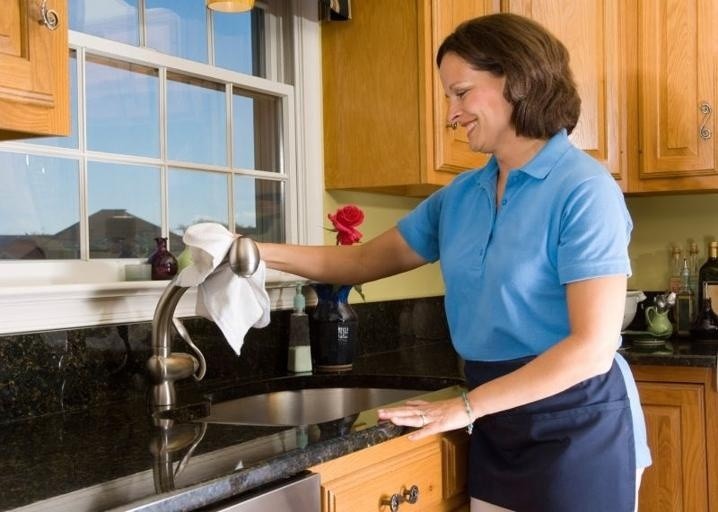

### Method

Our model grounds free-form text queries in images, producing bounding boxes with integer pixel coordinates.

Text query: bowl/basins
[620,290,645,332]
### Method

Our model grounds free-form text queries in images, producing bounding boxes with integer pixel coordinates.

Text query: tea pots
[645,306,674,339]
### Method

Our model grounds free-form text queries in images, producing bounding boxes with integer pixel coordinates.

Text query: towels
[172,221,272,360]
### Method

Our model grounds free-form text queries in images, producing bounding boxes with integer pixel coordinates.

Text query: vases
[307,281,357,371]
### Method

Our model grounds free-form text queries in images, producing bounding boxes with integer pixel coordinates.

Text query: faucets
[144,235,261,407]
[148,407,209,494]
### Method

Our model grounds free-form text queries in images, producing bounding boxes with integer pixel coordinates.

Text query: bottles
[669,237,717,342]
[145,236,178,279]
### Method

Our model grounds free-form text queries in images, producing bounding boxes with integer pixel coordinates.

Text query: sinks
[191,384,440,443]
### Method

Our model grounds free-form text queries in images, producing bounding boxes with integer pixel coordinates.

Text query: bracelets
[462,392,475,435]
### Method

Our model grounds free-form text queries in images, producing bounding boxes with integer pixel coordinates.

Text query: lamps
[206,1,255,14]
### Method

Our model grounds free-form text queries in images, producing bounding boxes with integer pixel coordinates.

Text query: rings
[421,415,427,425]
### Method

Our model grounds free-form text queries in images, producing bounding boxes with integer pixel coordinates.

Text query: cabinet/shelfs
[633,378,710,512]
[0,1,72,142]
[322,1,507,199]
[310,434,447,511]
[447,426,469,512]
[507,0,623,204]
[624,0,718,200]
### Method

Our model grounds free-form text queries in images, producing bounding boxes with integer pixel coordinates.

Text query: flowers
[317,203,370,299]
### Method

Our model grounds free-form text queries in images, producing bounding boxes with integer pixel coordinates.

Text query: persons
[232,13,653,512]
